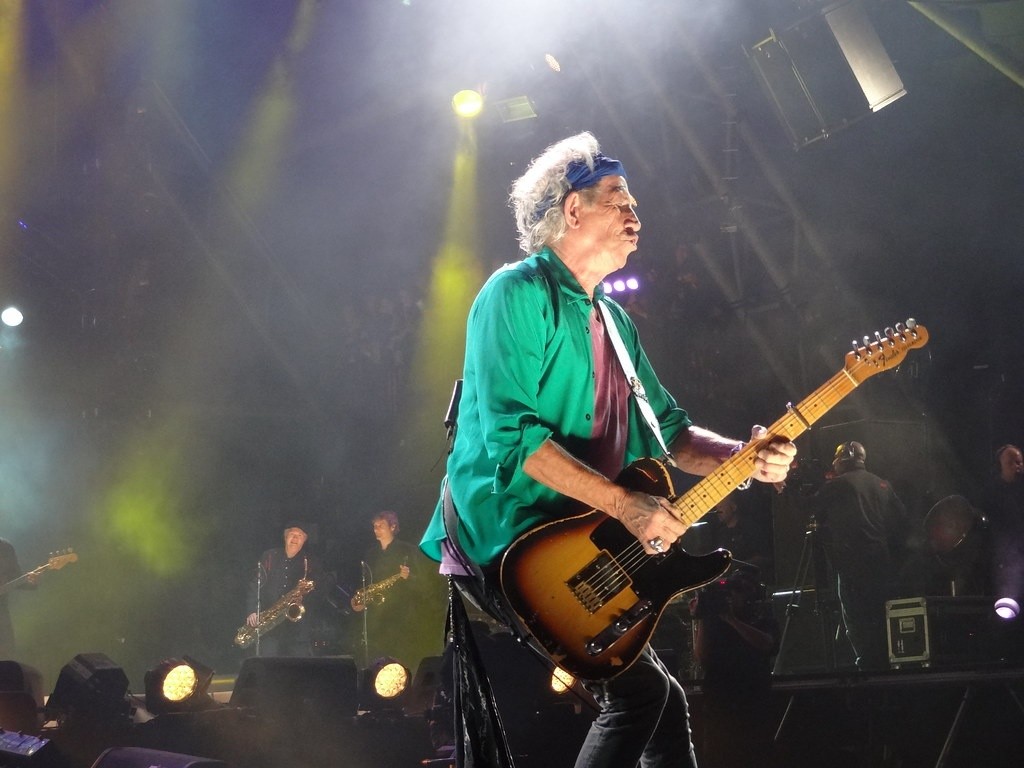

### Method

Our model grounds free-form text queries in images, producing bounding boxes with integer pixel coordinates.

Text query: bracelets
[730,441,752,490]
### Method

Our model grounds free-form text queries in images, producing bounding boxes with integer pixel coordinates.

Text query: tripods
[768,517,839,681]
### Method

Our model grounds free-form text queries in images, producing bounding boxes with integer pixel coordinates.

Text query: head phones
[841,441,854,461]
[995,445,1014,473]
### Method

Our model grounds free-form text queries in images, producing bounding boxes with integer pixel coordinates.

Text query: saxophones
[350,556,410,611]
[231,556,317,650]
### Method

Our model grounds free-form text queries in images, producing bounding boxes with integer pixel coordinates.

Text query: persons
[713,491,770,560]
[772,441,907,671]
[984,443,1024,669]
[0,538,39,661]
[689,566,780,768]
[417,131,798,768]
[245,515,327,657]
[352,510,427,667]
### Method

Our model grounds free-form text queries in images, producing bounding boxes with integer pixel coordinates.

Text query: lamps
[366,655,419,706]
[150,657,199,705]
[547,664,581,697]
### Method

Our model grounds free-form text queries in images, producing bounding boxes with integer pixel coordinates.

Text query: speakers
[230,656,358,720]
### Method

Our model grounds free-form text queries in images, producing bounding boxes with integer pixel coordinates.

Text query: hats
[283,518,308,536]
[374,511,399,532]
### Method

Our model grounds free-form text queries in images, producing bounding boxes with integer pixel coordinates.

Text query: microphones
[445,381,463,439]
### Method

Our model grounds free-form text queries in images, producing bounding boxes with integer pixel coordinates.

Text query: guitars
[495,315,928,685]
[0,546,79,597]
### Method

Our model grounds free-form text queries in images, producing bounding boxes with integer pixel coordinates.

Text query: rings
[651,538,663,552]
